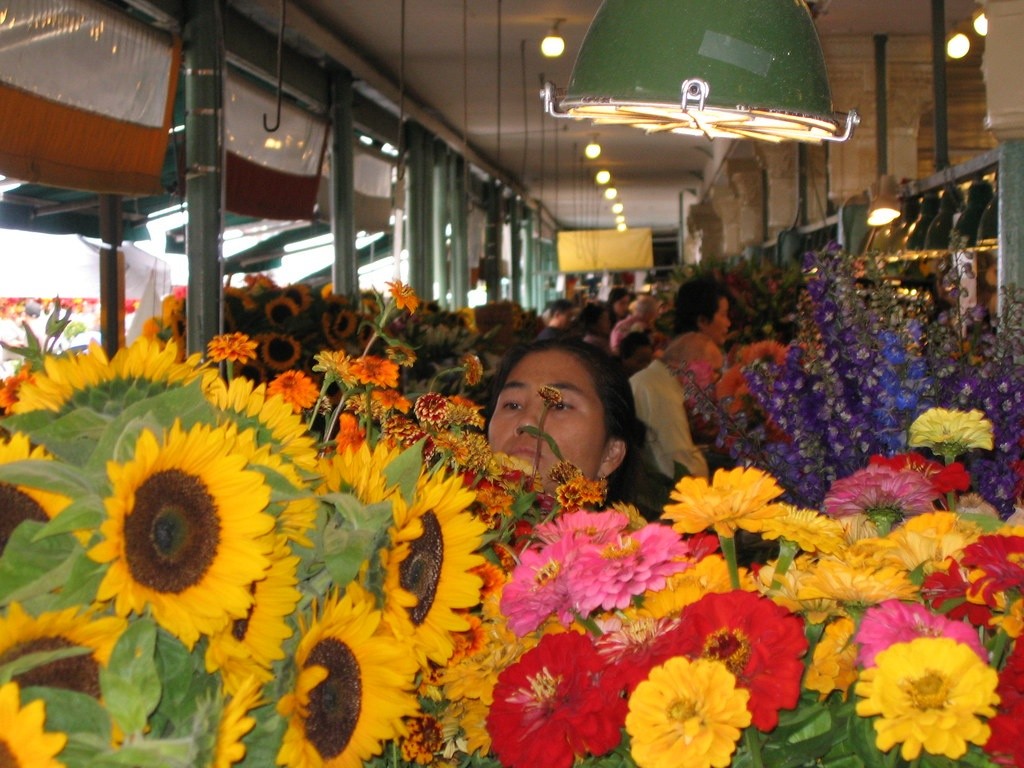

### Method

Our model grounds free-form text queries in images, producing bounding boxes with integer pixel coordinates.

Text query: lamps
[556,228,653,302]
[866,174,998,263]
[546,1,860,146]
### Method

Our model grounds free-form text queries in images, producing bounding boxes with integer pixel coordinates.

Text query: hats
[629,297,656,312]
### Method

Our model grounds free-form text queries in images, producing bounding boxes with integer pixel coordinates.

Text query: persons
[483,335,649,514]
[535,269,731,486]
